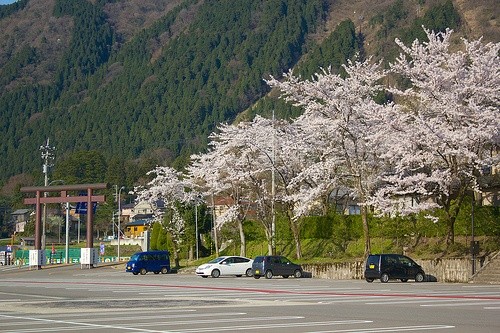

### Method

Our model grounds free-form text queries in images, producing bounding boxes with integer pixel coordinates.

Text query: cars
[195,255,255,278]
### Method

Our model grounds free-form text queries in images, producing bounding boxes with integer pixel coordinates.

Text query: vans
[364,253,426,283]
[251,255,304,279]
[125,250,170,275]
